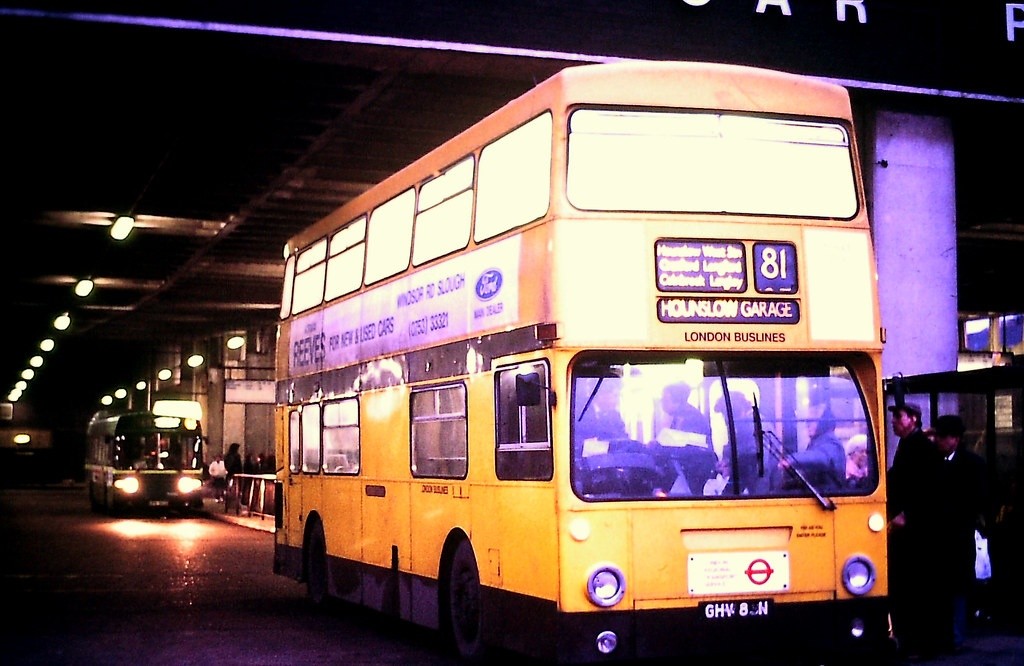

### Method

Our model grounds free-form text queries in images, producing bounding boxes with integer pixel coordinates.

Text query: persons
[960,400,986,430]
[931,416,987,655]
[662,381,716,452]
[887,402,944,660]
[778,403,847,493]
[844,434,867,479]
[714,391,762,496]
[583,377,630,445]
[209,443,242,504]
[244,453,276,474]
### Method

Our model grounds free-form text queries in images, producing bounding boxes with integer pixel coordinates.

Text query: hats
[887,404,922,419]
[928,415,967,437]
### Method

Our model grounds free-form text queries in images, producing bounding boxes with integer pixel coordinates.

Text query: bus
[82,408,209,516]
[273,60,899,666]
